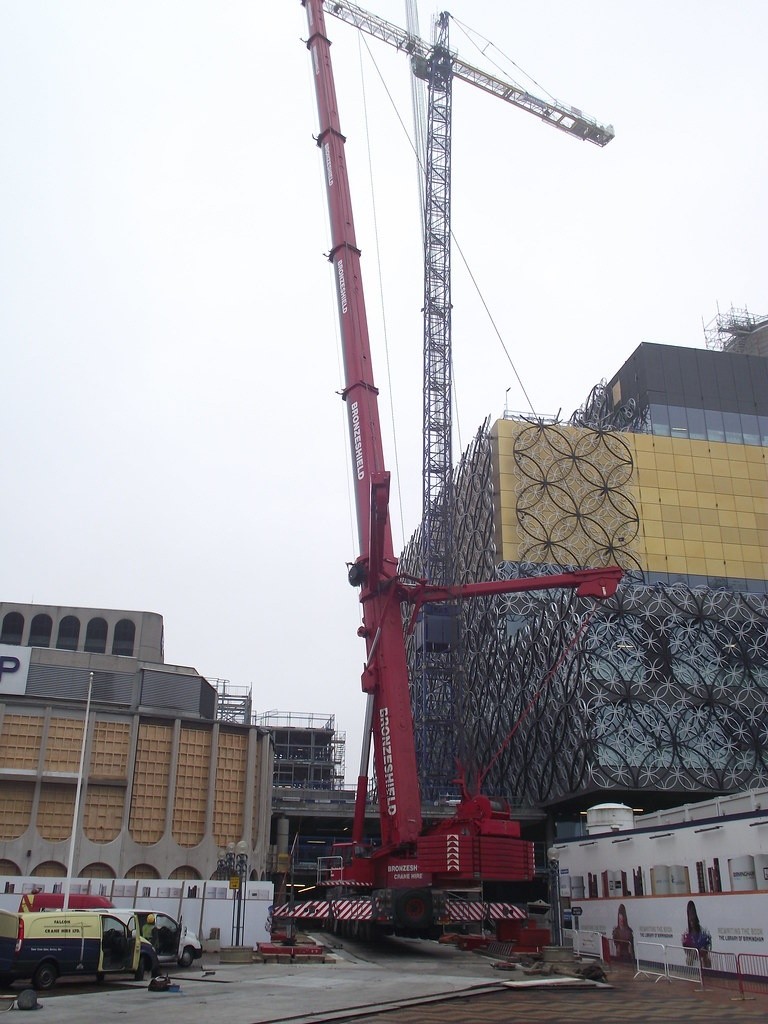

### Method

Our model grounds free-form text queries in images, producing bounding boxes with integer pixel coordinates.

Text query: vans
[0,910,161,988]
[16,892,116,915]
[55,907,203,968]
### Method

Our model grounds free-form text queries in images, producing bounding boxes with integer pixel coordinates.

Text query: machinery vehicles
[256,0,628,962]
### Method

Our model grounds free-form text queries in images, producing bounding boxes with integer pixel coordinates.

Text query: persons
[142,914,159,946]
[612,904,635,960]
[682,900,712,968]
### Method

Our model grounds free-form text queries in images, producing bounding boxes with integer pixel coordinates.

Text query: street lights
[547,845,565,949]
[218,840,250,947]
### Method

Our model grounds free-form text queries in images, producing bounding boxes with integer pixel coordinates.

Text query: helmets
[147,914,155,924]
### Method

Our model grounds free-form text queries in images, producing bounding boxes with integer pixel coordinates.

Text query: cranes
[319,0,619,798]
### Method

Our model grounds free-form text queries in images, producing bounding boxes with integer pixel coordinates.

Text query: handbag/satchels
[148,972,171,991]
